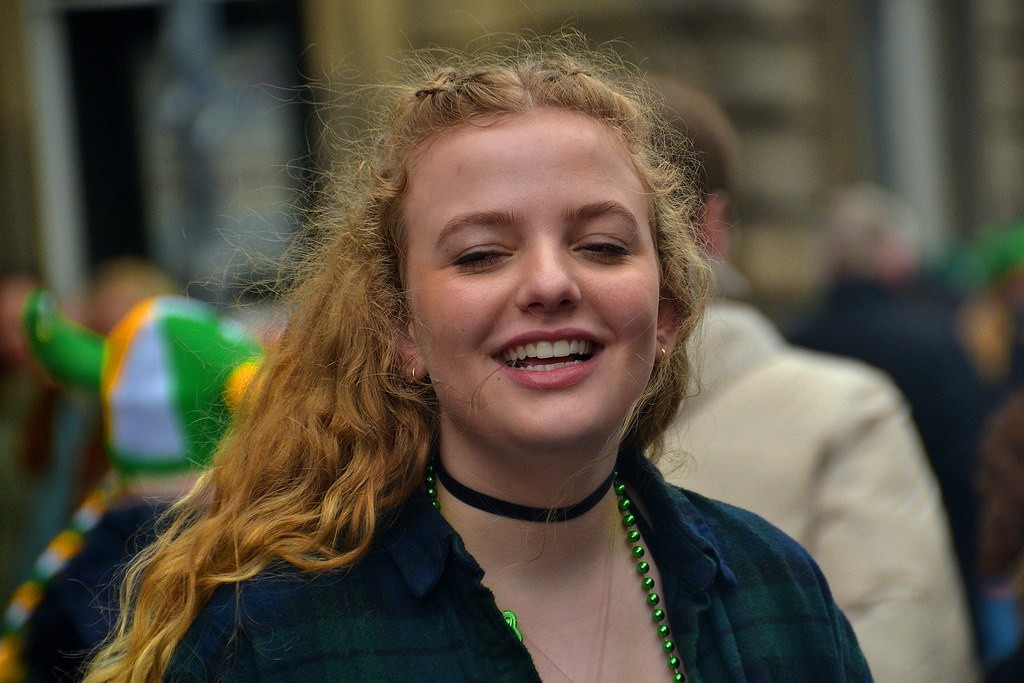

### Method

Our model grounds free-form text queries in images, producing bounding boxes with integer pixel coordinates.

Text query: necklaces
[434,449,615,522]
[425,456,686,683]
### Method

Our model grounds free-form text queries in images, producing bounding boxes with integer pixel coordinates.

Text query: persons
[24,289,268,683]
[786,185,991,568]
[978,391,1024,597]
[622,72,979,683]
[86,58,874,683]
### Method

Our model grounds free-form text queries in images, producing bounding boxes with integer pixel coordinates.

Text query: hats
[0,292,270,683]
